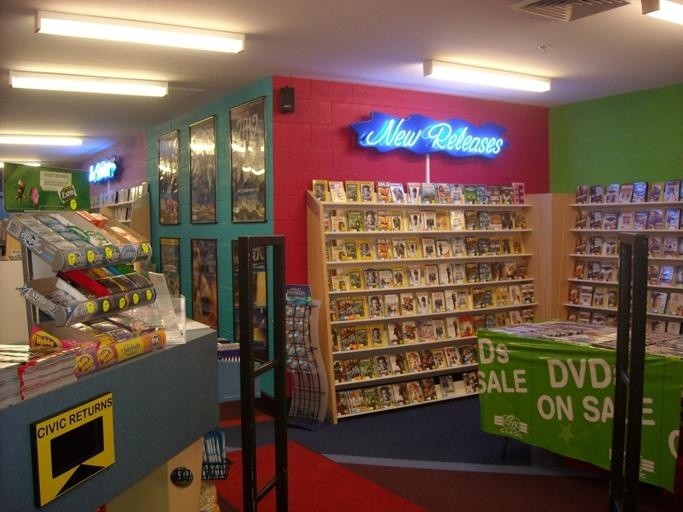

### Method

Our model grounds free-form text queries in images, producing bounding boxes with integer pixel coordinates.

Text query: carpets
[209,439,425,512]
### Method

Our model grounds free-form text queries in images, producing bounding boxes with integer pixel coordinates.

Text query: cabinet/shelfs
[89,191,151,243]
[557,199,683,336]
[306,188,540,425]
[6,206,167,377]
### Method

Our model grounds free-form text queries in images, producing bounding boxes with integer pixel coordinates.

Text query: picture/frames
[157,129,181,225]
[230,239,268,364]
[190,238,219,337]
[159,237,181,297]
[228,96,268,223]
[188,115,217,224]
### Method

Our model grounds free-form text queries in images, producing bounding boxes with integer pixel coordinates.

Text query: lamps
[33,8,246,53]
[0,134,82,148]
[423,59,551,92]
[641,0,683,26]
[8,69,170,99]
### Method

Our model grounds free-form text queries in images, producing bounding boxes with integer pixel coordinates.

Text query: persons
[365,212,376,228]
[371,328,381,344]
[377,357,388,374]
[369,297,381,315]
[361,243,371,256]
[192,248,203,322]
[362,186,371,201]
[366,271,377,286]
[381,387,391,404]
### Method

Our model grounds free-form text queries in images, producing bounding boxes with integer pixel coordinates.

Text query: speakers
[279,86,295,113]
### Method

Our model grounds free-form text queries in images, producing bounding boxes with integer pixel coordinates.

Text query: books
[0,342,81,410]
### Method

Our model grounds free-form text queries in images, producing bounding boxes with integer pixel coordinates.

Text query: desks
[477,318,683,502]
